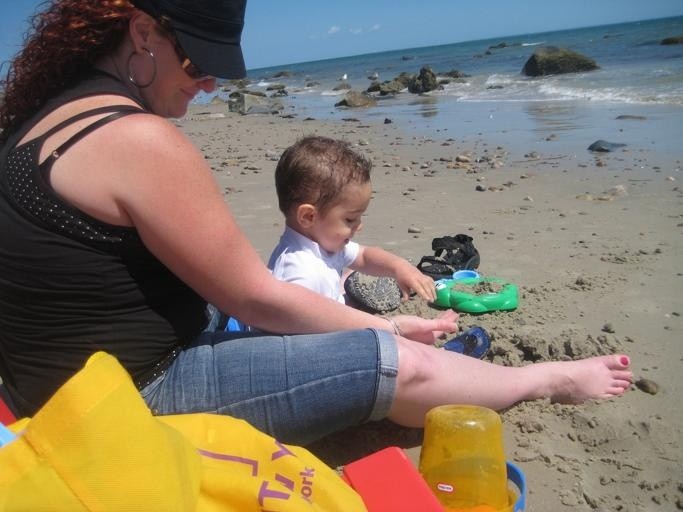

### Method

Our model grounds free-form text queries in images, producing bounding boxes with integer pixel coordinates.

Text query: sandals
[414,232,481,281]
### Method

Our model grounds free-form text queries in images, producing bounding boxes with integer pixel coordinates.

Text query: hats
[128,0,248,82]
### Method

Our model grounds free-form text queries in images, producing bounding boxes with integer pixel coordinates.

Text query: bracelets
[378,315,401,337]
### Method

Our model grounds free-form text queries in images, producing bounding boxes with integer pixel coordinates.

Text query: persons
[0,1,632,457]
[250,135,490,359]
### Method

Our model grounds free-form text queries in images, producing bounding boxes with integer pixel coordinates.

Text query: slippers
[440,325,492,363]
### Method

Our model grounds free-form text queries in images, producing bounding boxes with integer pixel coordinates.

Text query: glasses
[156,21,210,80]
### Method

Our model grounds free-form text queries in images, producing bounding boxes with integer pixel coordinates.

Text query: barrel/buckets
[419,403,526,512]
